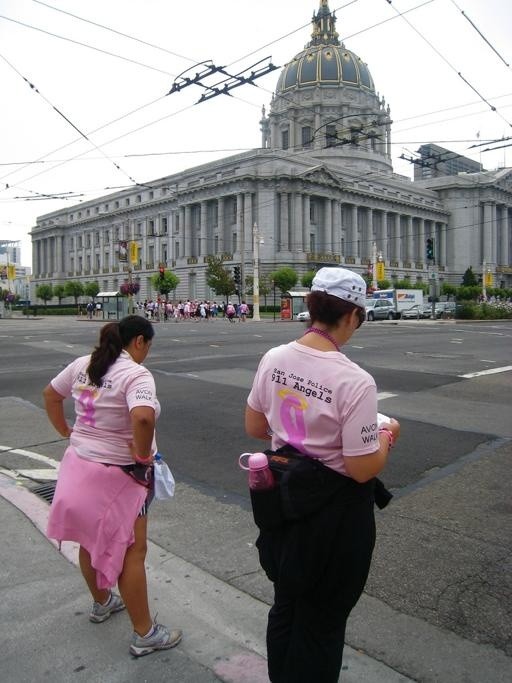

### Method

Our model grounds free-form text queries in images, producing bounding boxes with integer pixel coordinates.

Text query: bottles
[154,453,169,501]
[238,452,275,492]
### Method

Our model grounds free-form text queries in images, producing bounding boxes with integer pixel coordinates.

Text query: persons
[83,300,104,316]
[130,295,252,321]
[235,265,401,683]
[44,314,183,657]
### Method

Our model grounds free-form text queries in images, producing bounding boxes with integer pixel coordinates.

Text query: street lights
[370,240,382,290]
[481,260,492,301]
[97,210,134,315]
[251,221,265,321]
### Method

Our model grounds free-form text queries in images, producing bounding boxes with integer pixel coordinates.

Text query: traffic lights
[426,237,433,259]
[233,266,241,284]
[160,266,165,280]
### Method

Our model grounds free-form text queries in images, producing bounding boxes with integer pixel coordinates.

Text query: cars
[402,303,428,320]
[297,310,310,323]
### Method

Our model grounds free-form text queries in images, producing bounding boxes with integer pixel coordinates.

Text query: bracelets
[378,427,394,452]
[134,452,153,463]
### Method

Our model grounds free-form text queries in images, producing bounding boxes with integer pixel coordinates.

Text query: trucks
[373,288,424,318]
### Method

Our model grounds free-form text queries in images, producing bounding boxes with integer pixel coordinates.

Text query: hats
[310,264,367,312]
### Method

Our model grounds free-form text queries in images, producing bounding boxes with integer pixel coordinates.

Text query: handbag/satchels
[250,446,326,534]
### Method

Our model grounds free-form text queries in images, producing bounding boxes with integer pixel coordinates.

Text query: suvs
[424,301,457,318]
[364,297,401,322]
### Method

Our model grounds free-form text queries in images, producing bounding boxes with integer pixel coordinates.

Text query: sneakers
[128,625,184,655]
[90,592,128,624]
